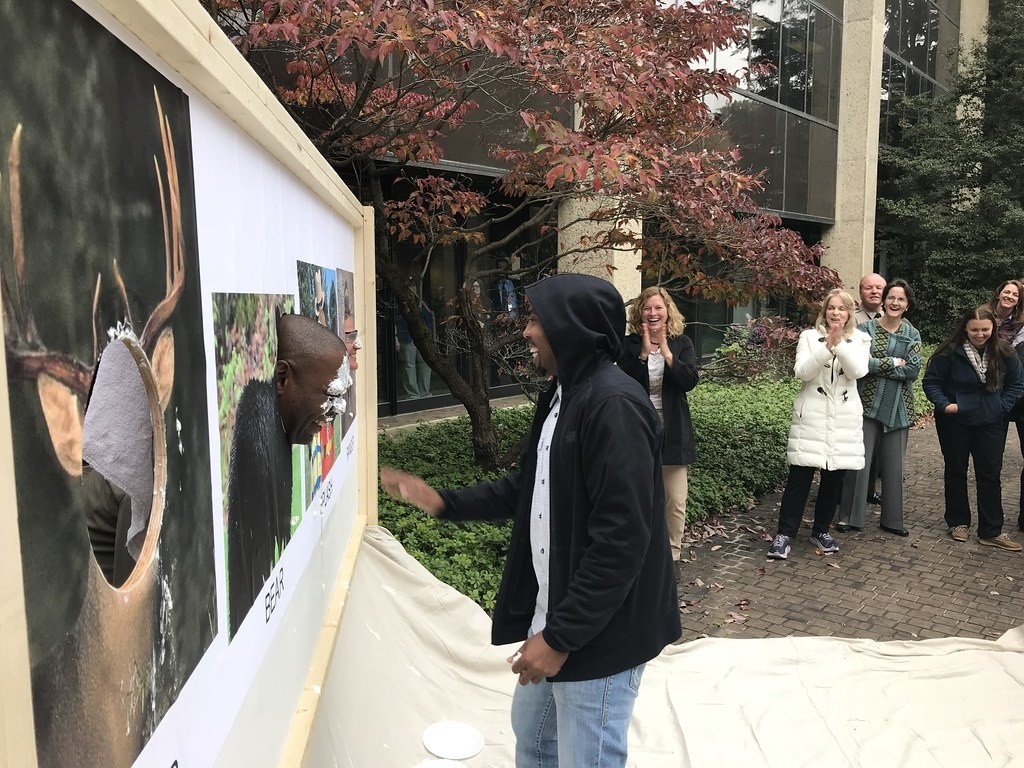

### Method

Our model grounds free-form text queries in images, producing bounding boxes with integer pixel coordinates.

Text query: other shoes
[866,492,884,504]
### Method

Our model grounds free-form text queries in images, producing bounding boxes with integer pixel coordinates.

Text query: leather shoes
[880,524,909,536]
[837,522,853,532]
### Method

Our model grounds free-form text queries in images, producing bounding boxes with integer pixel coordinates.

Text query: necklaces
[650,335,668,348]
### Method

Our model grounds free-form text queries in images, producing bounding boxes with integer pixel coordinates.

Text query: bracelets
[395,335,398,337]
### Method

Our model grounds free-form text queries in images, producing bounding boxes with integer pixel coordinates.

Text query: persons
[472,279,492,329]
[767,288,870,559]
[380,273,683,768]
[922,308,1023,551]
[345,306,362,370]
[394,278,435,400]
[490,258,522,386]
[852,272,887,504]
[618,286,699,585]
[836,279,922,537]
[977,280,1023,531]
[277,314,349,446]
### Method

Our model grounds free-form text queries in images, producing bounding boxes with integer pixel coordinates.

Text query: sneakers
[768,534,792,560]
[951,524,969,541]
[809,530,839,552]
[980,532,1022,552]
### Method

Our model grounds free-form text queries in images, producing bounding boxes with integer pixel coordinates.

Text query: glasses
[342,330,361,342]
